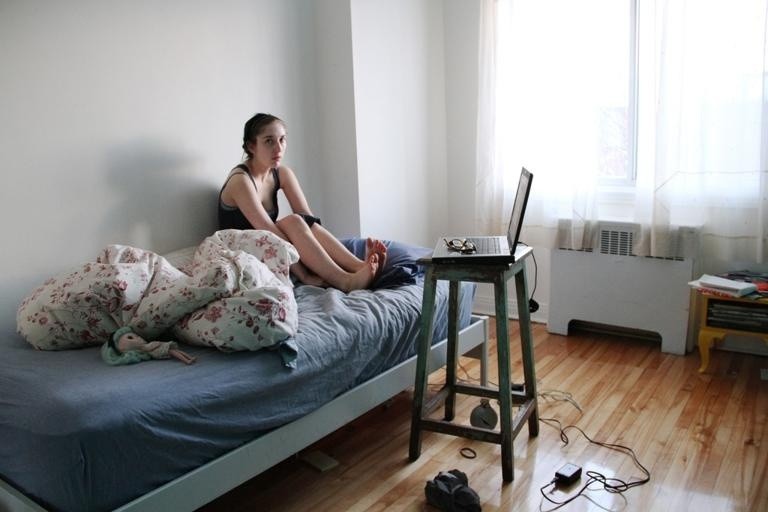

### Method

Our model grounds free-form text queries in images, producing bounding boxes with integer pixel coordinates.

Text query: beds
[0,239,492,512]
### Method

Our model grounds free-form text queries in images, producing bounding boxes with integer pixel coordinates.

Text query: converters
[555,463,582,483]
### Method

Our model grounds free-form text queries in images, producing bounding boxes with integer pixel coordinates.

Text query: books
[688,269,768,328]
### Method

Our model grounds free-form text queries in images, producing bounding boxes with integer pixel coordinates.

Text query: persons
[101,325,196,366]
[219,113,387,294]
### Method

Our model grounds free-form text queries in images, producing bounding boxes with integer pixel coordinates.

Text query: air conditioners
[542,217,703,359]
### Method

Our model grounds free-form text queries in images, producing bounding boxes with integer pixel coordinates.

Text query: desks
[686,276,768,375]
[402,236,543,490]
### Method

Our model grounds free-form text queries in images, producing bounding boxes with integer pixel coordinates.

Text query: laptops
[432,166,533,261]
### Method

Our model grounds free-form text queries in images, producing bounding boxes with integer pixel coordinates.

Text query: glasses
[442,236,477,254]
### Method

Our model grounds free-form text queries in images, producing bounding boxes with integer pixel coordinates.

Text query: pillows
[326,235,439,288]
